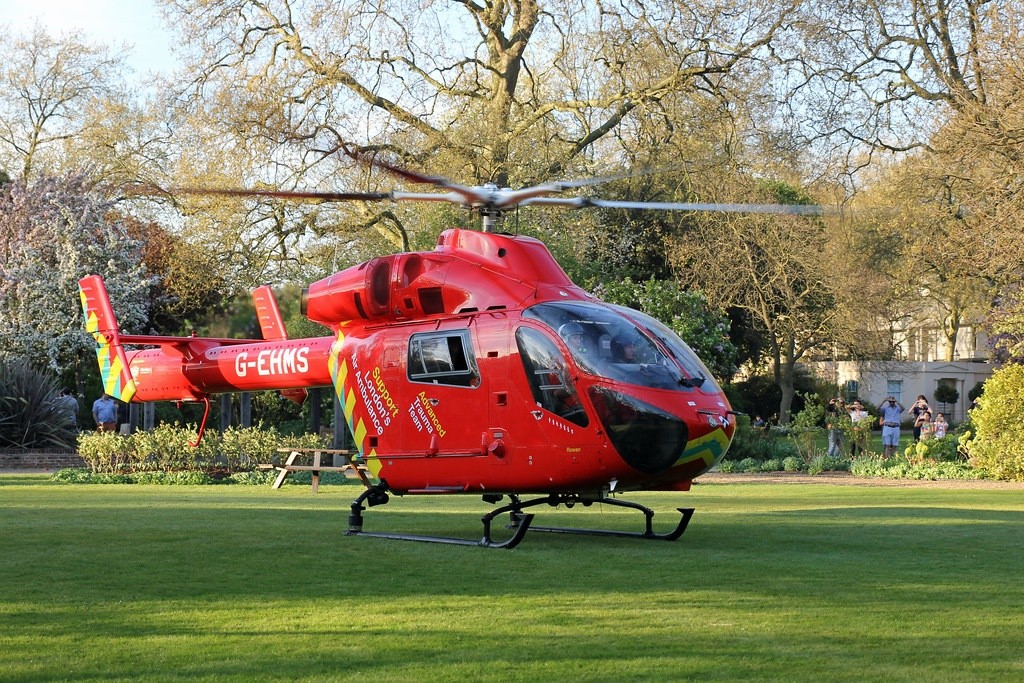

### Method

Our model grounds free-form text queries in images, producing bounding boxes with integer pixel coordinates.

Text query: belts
[885,424,899,427]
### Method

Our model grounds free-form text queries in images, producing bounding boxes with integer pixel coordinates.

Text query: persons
[611,333,636,364]
[879,397,905,459]
[550,322,610,423]
[828,397,847,456]
[845,400,868,457]
[55,390,79,430]
[752,416,763,430]
[92,392,118,431]
[908,395,948,443]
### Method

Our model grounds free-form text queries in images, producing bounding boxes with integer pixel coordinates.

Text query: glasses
[889,401,895,403]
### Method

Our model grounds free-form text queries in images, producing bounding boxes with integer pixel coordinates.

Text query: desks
[272,446,373,493]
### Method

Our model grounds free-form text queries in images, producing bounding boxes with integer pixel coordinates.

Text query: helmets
[610,334,636,358]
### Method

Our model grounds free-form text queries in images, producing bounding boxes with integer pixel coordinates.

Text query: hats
[888,396,897,401]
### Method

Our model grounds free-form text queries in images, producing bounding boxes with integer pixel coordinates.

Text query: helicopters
[77,154,858,549]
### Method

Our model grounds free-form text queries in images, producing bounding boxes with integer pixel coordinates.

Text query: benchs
[273,463,347,472]
[342,463,368,472]
